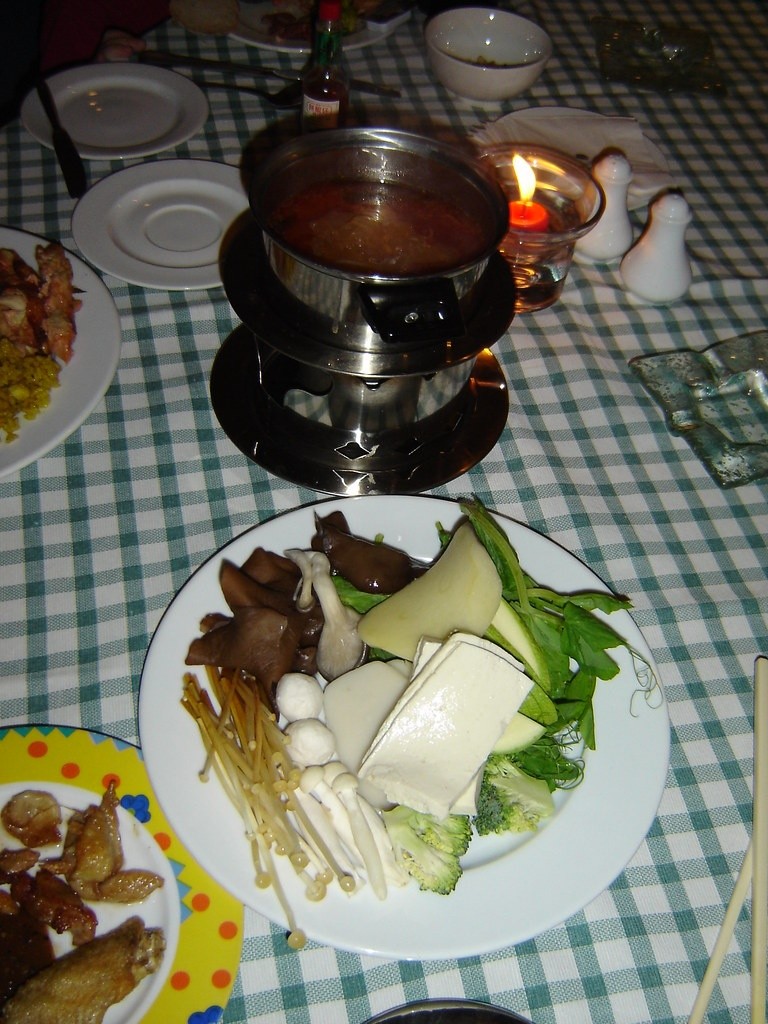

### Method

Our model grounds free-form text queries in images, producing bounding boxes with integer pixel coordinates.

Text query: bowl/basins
[425,8,552,108]
[256,127,509,350]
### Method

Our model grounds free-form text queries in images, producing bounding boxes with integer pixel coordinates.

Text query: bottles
[301,2,350,132]
[572,153,632,264]
[620,193,693,306]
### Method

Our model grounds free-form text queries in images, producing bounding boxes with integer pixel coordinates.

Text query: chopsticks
[688,657,768,1024]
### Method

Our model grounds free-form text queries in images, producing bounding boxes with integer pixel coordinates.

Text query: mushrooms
[299,761,412,899]
[284,548,370,681]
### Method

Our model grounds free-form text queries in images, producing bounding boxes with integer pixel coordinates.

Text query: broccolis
[382,806,473,895]
[469,755,555,836]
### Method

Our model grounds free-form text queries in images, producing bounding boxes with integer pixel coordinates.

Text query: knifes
[32,68,86,199]
[138,52,403,99]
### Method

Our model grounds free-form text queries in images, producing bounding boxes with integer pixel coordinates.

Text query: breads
[168,0,241,36]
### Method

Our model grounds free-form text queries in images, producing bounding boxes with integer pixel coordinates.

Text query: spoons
[197,82,303,107]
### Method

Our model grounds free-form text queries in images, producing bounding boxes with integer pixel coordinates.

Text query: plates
[0,724,243,1024]
[71,158,250,291]
[21,63,209,159]
[0,227,121,477]
[227,23,394,54]
[139,495,671,960]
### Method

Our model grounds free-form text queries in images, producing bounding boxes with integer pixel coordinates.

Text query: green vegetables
[331,491,635,791]
